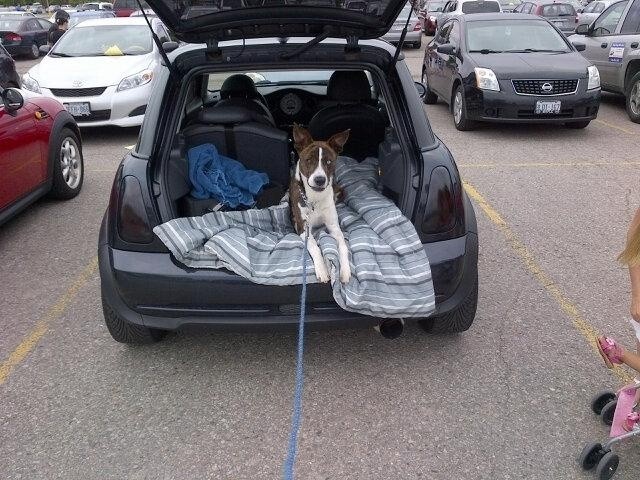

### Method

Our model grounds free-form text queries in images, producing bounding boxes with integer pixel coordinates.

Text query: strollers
[580,381,639,480]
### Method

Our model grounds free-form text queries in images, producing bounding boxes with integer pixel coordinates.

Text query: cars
[0,43,84,227]
[381,0,620,49]
[20,15,174,132]
[420,13,602,134]
[1,0,230,61]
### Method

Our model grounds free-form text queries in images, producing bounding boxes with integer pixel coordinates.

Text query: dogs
[283,122,352,286]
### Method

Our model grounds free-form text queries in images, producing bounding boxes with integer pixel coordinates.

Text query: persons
[595,335,640,374]
[21,5,71,50]
[617,205,640,323]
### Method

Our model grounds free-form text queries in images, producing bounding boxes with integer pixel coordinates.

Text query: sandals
[621,412,639,433]
[596,335,624,372]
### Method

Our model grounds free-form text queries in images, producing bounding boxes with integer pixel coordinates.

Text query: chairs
[213,74,273,125]
[310,70,386,162]
[182,109,290,184]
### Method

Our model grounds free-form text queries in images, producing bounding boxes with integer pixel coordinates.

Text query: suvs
[563,0,640,123]
[97,1,478,345]
